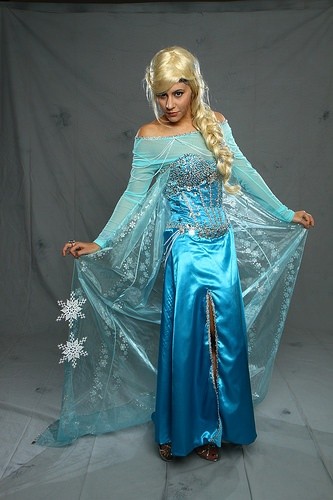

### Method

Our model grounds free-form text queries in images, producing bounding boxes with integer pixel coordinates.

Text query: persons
[33,46,316,461]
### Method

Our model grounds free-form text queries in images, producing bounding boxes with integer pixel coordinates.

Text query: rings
[68,240,75,246]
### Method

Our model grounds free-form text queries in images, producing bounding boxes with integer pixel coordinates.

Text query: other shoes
[158,441,181,462]
[194,442,220,462]
